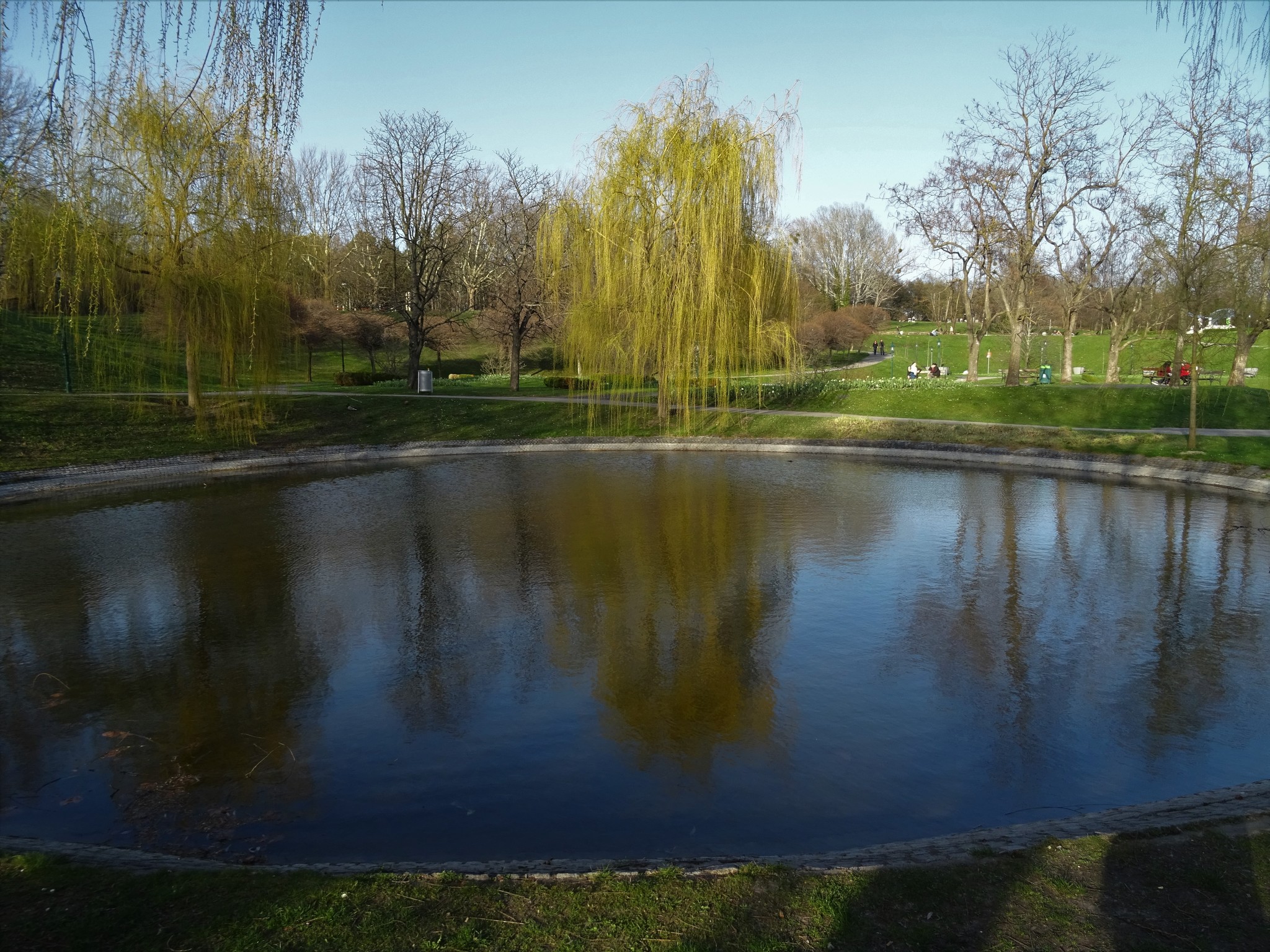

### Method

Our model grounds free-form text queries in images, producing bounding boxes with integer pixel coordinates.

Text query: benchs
[1001,367,1041,384]
[917,369,946,380]
[1140,366,1225,386]
[1080,368,1095,375]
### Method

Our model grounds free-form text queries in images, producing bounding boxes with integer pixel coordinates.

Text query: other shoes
[1182,383,1186,386]
[1162,383,1169,386]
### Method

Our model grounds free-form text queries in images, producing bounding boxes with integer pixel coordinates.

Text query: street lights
[936,337,942,367]
[930,345,933,366]
[888,341,896,378]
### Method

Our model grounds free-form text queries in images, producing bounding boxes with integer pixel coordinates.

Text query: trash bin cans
[417,370,432,392]
[1040,364,1052,383]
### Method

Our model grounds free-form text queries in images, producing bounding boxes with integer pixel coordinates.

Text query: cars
[1210,308,1234,318]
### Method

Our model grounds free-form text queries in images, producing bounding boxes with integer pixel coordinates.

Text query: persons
[949,326,953,335]
[1180,360,1191,386]
[1157,360,1171,385]
[1052,330,1061,335]
[896,326,899,334]
[928,363,940,378]
[907,362,922,379]
[879,339,885,355]
[873,340,878,355]
